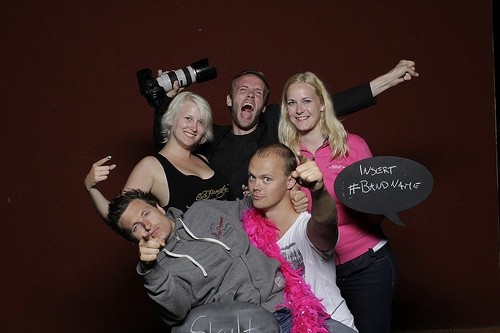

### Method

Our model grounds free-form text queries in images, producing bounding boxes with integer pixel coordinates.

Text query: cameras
[137,58,218,109]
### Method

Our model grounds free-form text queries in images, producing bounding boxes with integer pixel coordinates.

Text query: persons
[278,71,400,332]
[235,143,360,332]
[148,59,419,201]
[105,188,359,332]
[84,90,233,245]
